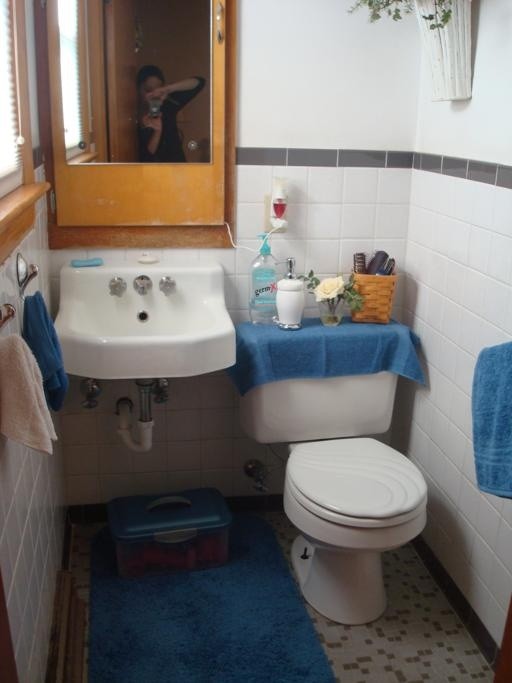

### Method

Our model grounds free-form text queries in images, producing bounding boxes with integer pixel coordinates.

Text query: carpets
[84,491,344,683]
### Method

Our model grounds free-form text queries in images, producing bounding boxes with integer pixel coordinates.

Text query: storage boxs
[105,484,234,577]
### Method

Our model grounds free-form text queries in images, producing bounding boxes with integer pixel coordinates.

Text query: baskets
[348,269,399,325]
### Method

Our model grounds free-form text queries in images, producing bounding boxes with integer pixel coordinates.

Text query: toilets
[235,321,429,627]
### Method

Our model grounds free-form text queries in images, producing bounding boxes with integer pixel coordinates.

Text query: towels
[223,313,429,396]
[2,332,60,455]
[18,291,70,412]
[470,340,511,500]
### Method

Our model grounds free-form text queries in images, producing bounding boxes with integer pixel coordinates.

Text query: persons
[132,62,207,161]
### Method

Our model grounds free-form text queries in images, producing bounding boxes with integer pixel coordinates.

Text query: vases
[316,299,349,328]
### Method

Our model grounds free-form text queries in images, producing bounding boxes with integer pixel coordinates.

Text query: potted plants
[346,0,475,104]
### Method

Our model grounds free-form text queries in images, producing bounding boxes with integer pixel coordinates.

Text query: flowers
[298,266,362,313]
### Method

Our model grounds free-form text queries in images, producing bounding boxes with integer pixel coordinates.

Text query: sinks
[53,262,239,380]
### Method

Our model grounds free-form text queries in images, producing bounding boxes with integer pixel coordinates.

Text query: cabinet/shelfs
[46,0,227,226]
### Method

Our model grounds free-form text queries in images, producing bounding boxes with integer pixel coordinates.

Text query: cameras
[148,97,161,117]
